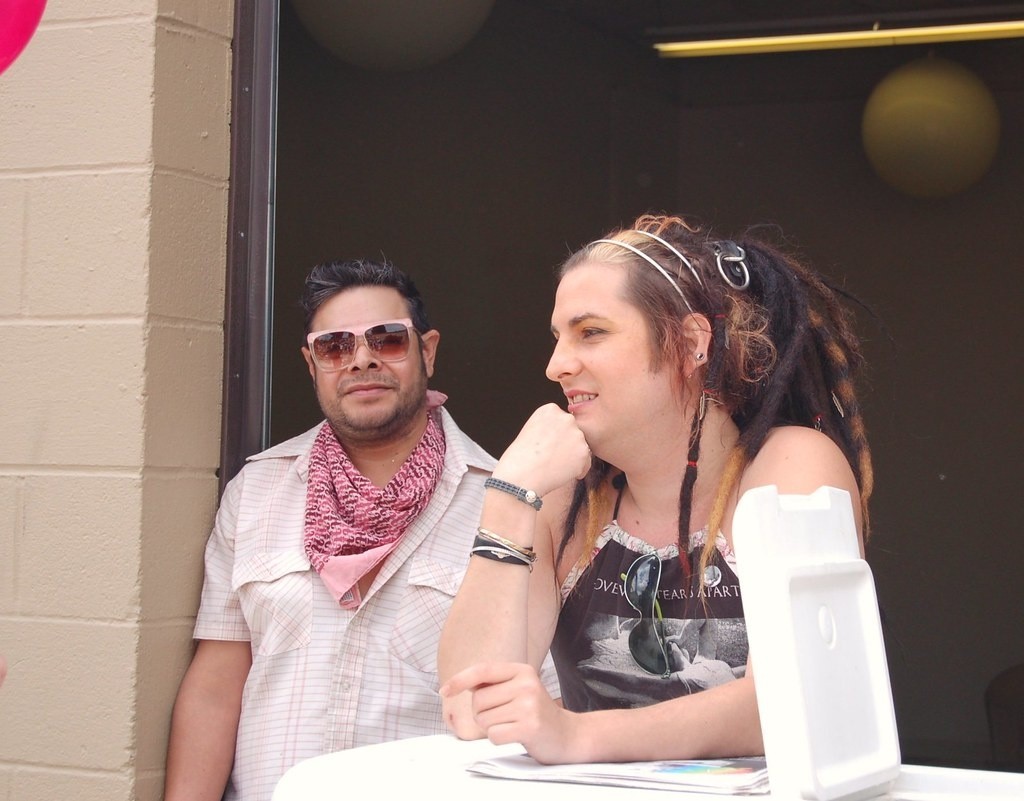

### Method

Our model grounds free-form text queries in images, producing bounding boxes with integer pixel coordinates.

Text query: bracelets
[484,477,543,510]
[469,527,538,573]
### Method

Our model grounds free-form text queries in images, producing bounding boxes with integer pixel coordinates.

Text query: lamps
[860,54,1001,196]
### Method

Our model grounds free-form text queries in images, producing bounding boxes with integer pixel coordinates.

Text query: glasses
[623,554,672,679]
[306,318,415,373]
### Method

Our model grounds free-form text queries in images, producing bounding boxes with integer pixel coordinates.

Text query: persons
[437,209,877,765]
[158,255,499,801]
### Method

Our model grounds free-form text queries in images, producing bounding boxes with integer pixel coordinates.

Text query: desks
[271,735,1024,801]
